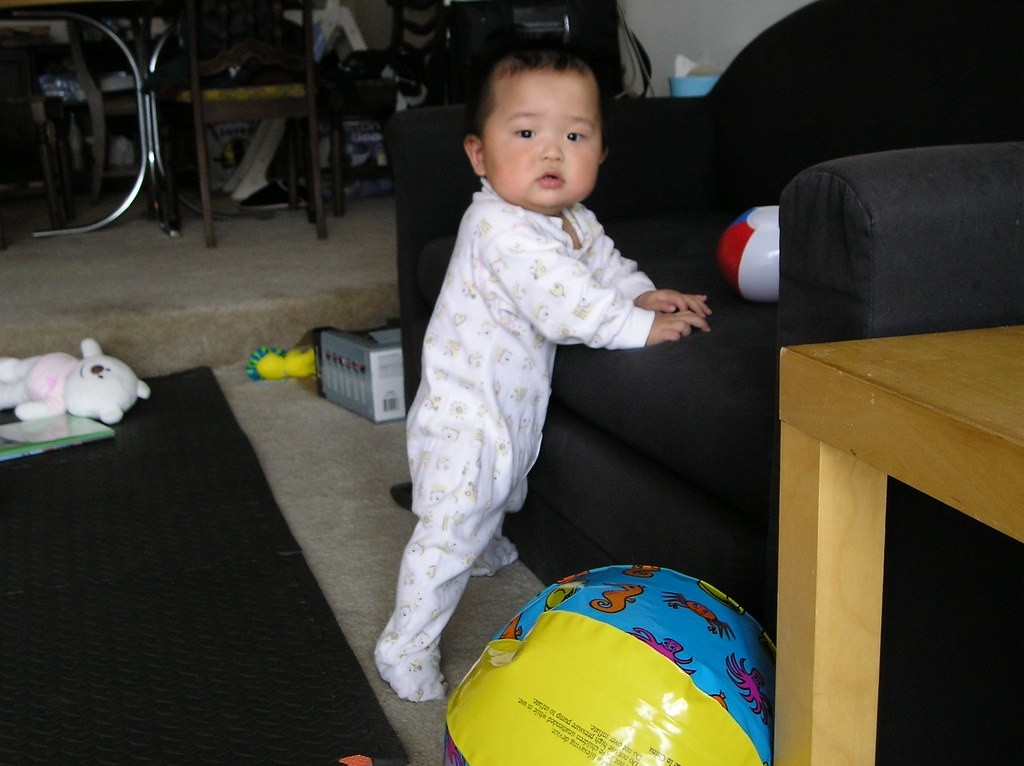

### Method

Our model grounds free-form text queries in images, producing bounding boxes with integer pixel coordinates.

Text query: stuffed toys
[0,337,150,424]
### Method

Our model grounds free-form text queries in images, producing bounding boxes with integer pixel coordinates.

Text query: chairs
[0,0,446,249]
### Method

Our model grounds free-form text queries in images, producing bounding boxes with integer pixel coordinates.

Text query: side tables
[770,325,1023,766]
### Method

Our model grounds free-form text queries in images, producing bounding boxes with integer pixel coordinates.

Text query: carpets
[0,364,409,766]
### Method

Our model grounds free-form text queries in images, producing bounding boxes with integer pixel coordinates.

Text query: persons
[373,36,712,704]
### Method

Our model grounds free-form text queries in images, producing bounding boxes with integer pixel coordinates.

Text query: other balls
[442,564,777,766]
[719,205,779,303]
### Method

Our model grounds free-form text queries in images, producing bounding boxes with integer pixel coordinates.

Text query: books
[0,413,115,462]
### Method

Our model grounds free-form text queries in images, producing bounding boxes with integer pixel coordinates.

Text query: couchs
[381,0,1024,765]
